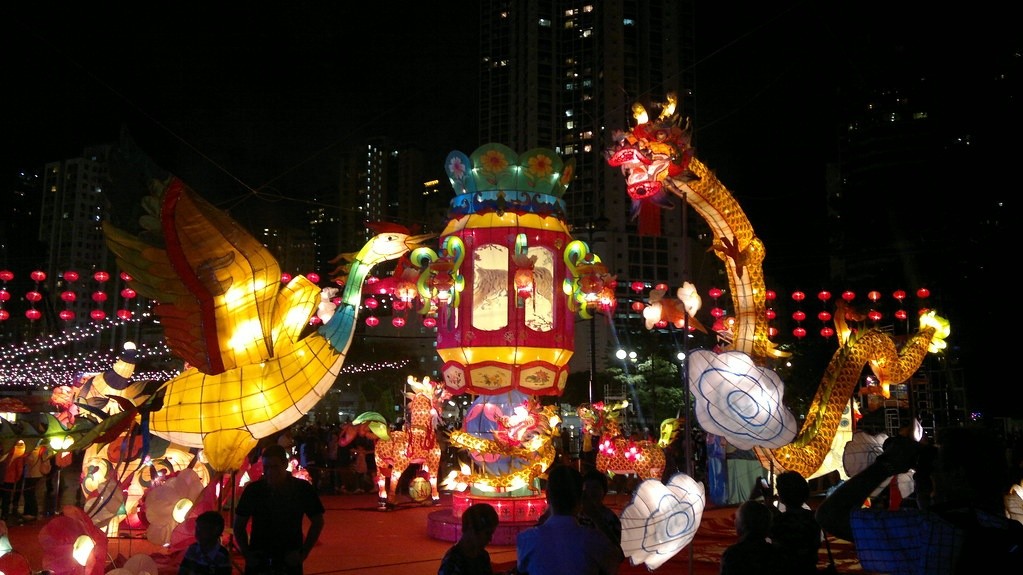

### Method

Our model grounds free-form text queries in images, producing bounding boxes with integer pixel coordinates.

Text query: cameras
[883,437,938,471]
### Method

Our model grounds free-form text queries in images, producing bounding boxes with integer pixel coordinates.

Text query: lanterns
[842,290,859,336]
[792,291,807,339]
[59,270,80,323]
[511,251,538,310]
[25,269,47,323]
[708,287,727,333]
[868,287,932,320]
[652,282,699,332]
[90,270,109,323]
[391,259,458,330]
[363,277,384,327]
[576,260,645,316]
[0,268,14,320]
[764,290,779,337]
[278,270,344,328]
[117,272,136,321]
[816,290,835,340]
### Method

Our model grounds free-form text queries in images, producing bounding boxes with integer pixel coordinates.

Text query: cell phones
[760,476,771,494]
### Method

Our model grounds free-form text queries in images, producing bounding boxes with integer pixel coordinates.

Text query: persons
[536,468,622,545]
[177,509,233,575]
[258,411,418,497]
[551,402,713,494]
[747,469,823,575]
[0,415,88,526]
[436,503,510,575]
[233,443,328,575]
[813,406,1023,575]
[716,499,797,575]
[513,464,625,575]
